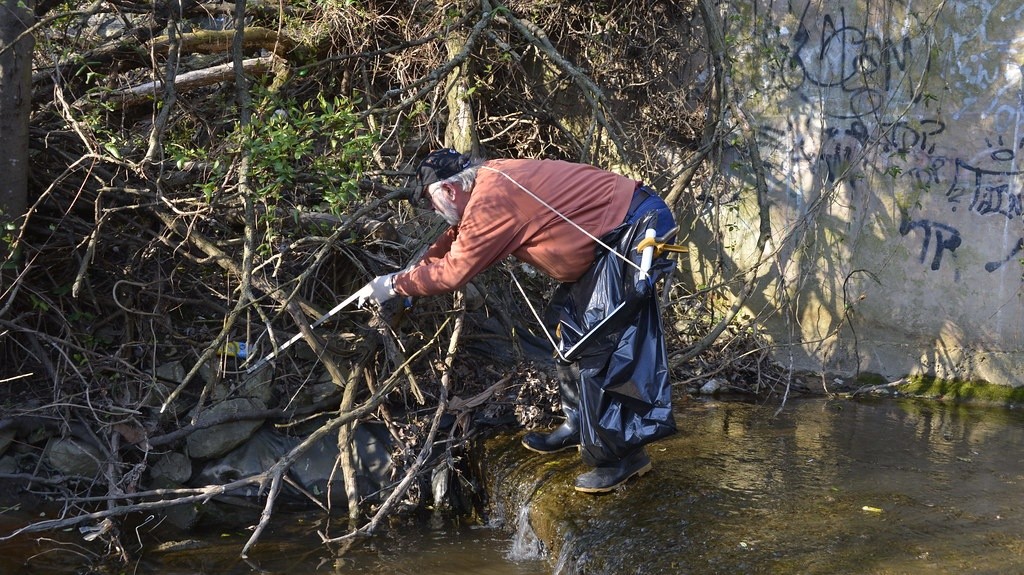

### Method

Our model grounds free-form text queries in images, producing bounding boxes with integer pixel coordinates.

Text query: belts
[622,187,654,219]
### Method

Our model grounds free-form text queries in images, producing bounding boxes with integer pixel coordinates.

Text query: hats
[413,148,471,202]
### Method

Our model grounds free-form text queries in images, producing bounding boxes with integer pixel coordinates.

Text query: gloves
[357,274,398,309]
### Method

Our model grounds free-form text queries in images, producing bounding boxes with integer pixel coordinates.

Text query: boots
[521,363,581,453]
[574,443,654,493]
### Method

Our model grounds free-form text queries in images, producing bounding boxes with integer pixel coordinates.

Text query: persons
[357,149,674,493]
